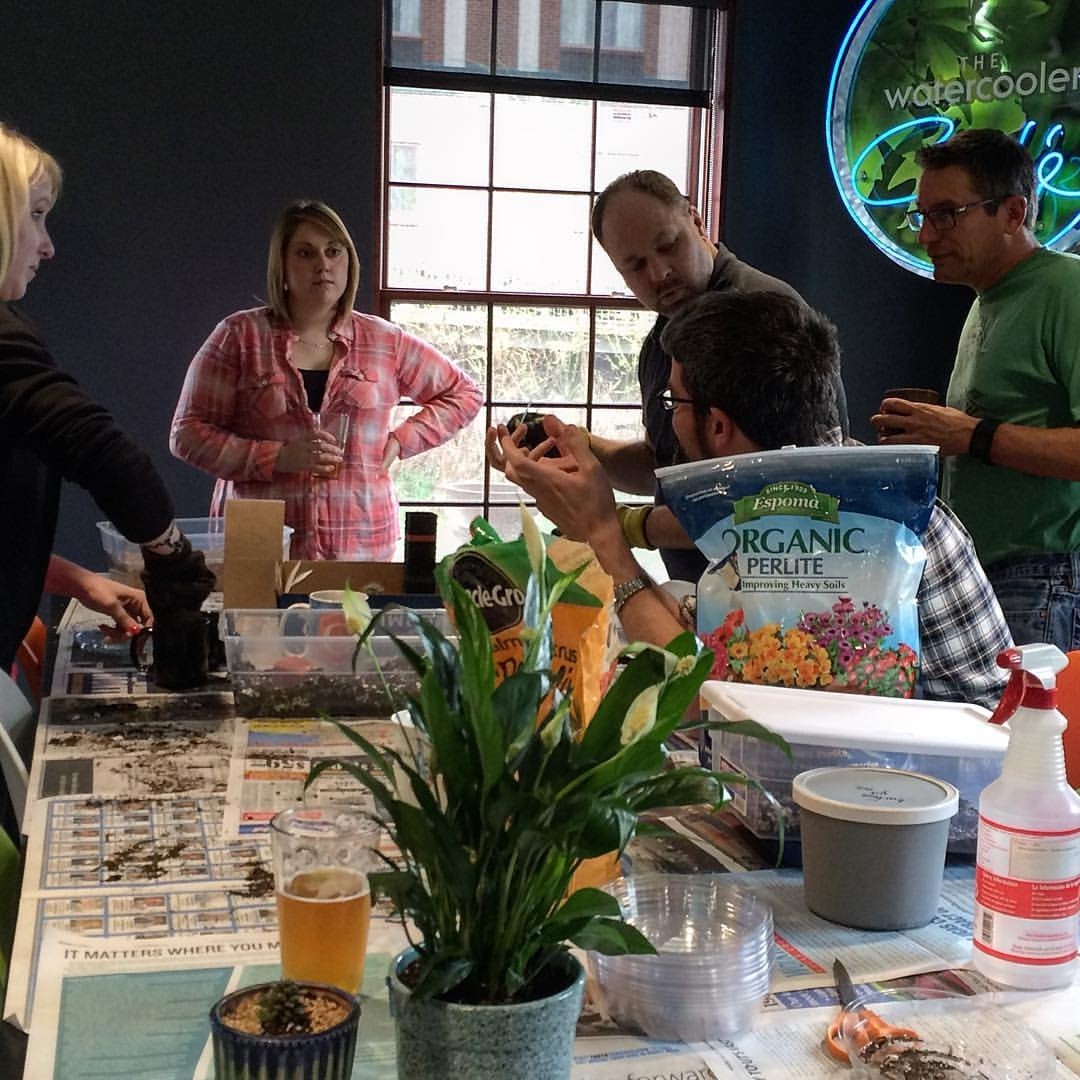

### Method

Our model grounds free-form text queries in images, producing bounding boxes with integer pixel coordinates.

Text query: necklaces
[294,329,338,349]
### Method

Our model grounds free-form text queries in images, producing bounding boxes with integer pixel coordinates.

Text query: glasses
[657,386,729,412]
[905,192,1033,231]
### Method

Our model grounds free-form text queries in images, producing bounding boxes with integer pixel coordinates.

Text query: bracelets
[576,425,594,447]
[611,574,649,614]
[145,520,182,556]
[622,502,658,551]
[968,416,1005,463]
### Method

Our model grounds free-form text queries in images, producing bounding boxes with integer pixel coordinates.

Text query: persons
[490,278,1021,752]
[480,168,851,587]
[166,201,484,567]
[870,129,1079,690]
[0,129,188,759]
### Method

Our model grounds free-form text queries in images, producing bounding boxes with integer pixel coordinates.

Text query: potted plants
[210,975,361,1080]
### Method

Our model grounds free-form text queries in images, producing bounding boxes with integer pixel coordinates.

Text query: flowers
[313,493,794,1009]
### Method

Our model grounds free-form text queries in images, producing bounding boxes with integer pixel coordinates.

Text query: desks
[3,597,1080,1079]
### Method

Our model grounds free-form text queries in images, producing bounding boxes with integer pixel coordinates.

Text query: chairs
[17,617,46,700]
[0,826,20,986]
[0,666,33,839]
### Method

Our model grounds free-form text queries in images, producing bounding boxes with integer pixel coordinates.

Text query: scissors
[826,958,921,1062]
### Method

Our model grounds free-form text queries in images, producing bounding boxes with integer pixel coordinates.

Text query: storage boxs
[704,683,1011,855]
[215,604,463,718]
[224,499,285,611]
[94,517,294,591]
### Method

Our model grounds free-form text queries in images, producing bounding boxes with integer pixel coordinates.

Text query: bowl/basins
[836,988,1056,1079]
[70,617,133,653]
[583,873,778,1040]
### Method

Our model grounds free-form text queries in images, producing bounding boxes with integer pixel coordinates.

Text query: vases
[387,935,587,1078]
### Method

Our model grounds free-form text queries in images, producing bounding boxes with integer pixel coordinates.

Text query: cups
[129,613,217,690]
[310,412,350,482]
[792,766,962,932]
[269,806,381,999]
[280,590,369,670]
[879,388,939,437]
